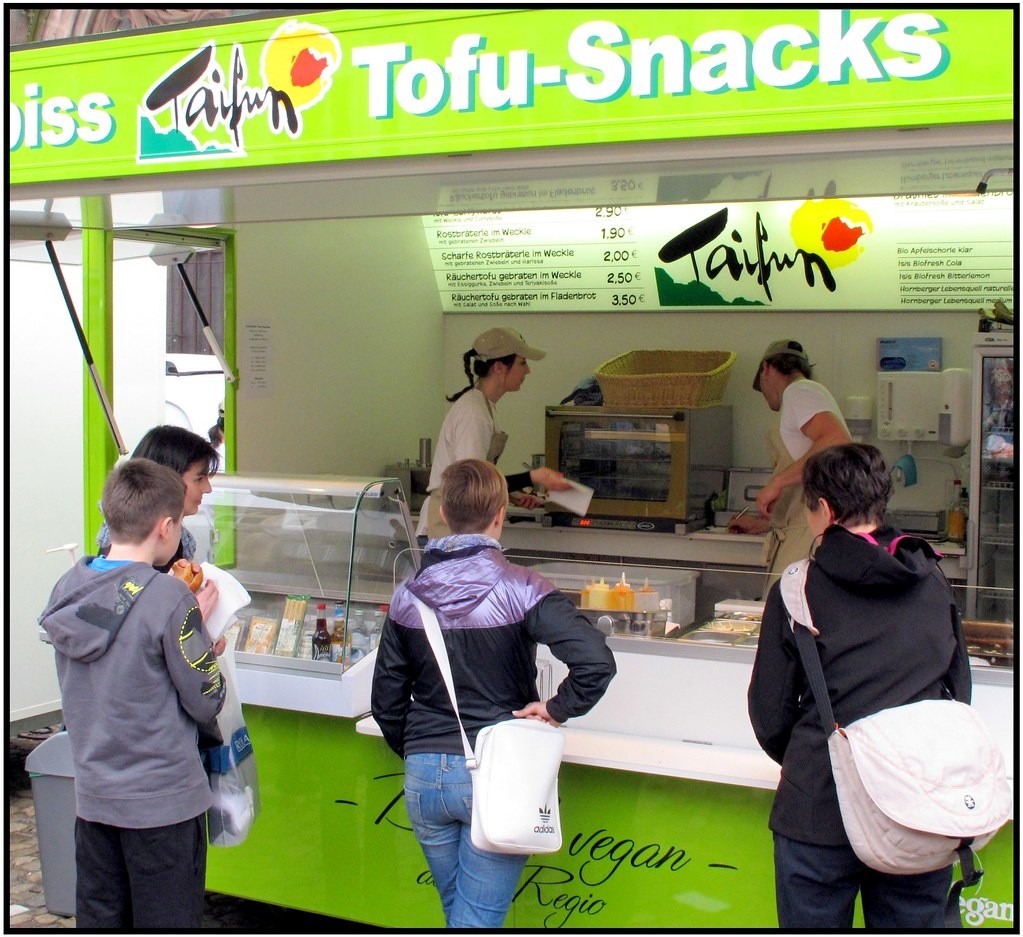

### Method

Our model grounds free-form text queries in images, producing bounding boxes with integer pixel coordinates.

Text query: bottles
[330,600,350,670]
[350,609,369,667]
[369,611,380,652]
[375,605,390,649]
[579,572,660,611]
[311,603,331,662]
[946,478,969,541]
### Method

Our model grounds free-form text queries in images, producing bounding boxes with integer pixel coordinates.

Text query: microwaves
[544,404,734,534]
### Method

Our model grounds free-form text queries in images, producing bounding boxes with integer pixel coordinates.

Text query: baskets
[594,348,736,409]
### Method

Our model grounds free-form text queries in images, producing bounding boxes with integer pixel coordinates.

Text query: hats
[471,327,547,362]
[752,339,809,392]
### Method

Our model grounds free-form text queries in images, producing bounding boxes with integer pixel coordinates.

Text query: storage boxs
[523,558,701,632]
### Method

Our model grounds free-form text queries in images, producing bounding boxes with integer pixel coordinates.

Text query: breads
[170,558,203,593]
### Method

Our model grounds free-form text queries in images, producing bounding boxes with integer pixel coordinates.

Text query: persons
[98,425,220,578]
[748,444,971,927]
[413,326,572,550]
[726,340,851,603]
[371,460,615,930]
[37,458,226,928]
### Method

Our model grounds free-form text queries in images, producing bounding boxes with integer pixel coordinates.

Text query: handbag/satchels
[207,638,261,848]
[466,717,567,859]
[781,559,1013,875]
